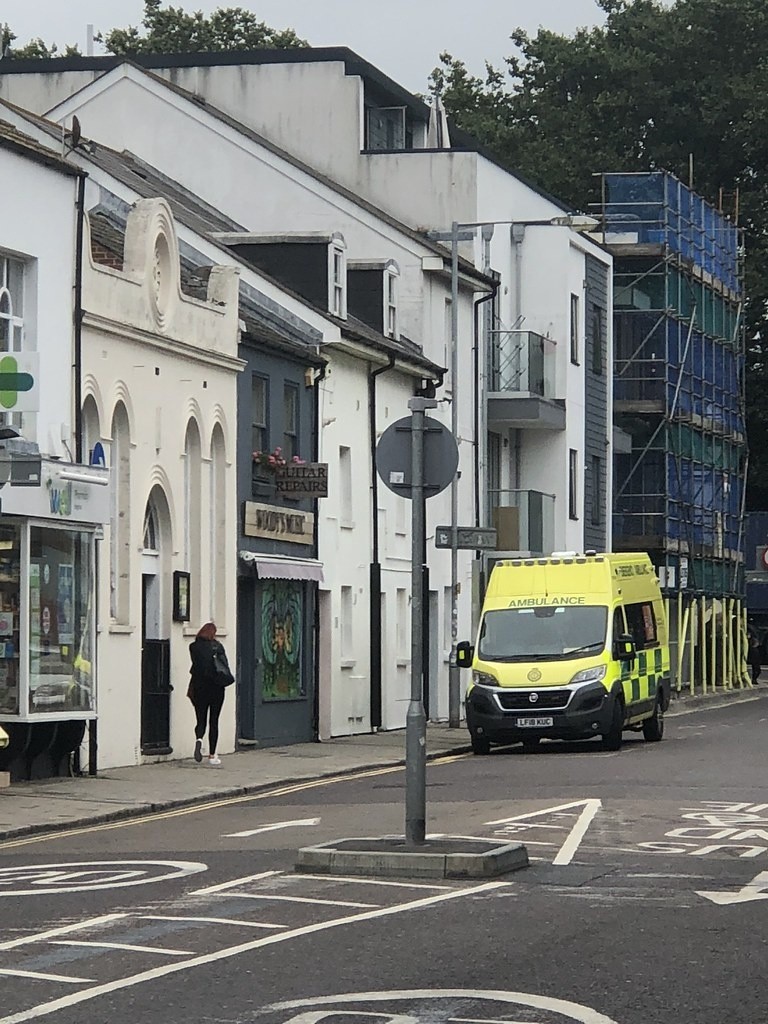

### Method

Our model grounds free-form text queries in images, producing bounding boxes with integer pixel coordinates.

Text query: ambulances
[455,550,670,755]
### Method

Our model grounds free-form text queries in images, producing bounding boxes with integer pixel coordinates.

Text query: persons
[747,638,762,685]
[186,623,228,765]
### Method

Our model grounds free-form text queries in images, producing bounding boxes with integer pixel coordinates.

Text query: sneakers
[208,755,221,764]
[193,739,203,763]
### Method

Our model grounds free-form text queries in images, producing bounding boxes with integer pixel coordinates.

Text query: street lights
[448,215,603,733]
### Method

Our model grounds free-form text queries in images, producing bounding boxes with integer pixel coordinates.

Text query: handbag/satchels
[213,640,233,687]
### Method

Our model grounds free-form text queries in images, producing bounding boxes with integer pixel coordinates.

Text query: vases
[253,461,275,483]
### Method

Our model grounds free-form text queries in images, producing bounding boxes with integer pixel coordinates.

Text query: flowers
[252,447,305,475]
